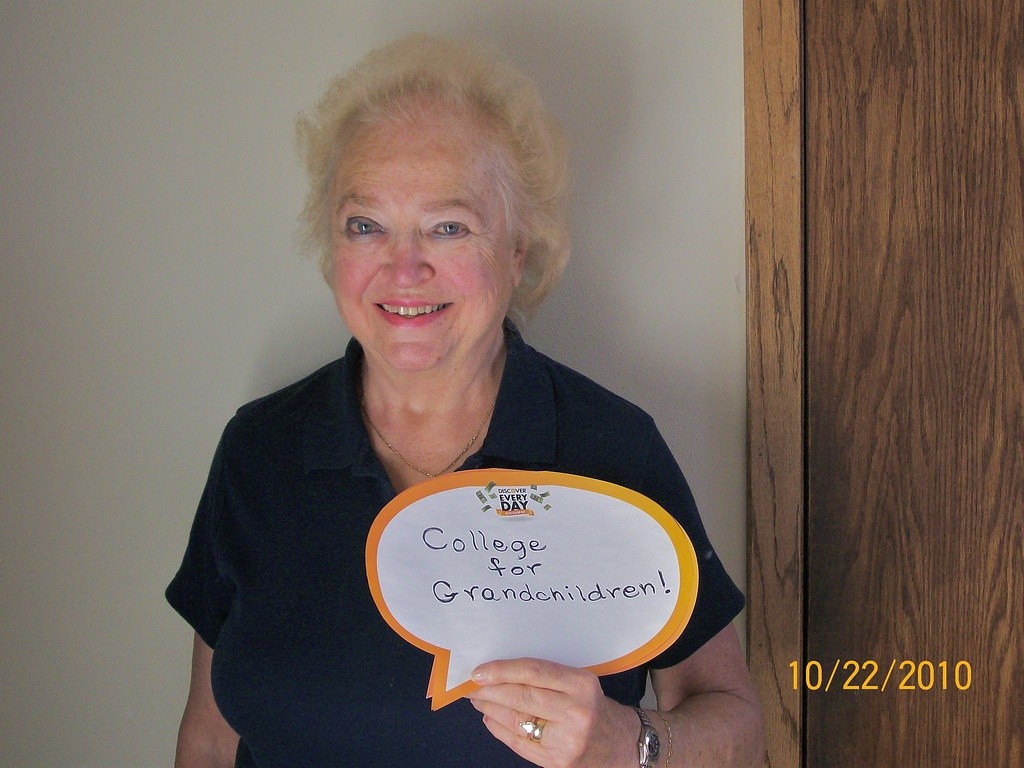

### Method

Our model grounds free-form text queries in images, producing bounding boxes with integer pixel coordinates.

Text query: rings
[520,715,547,742]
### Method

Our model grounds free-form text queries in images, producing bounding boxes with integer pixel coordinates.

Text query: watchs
[632,707,661,768]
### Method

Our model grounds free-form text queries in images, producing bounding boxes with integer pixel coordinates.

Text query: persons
[164,38,766,768]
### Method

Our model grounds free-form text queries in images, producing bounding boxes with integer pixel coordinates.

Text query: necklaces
[362,394,495,478]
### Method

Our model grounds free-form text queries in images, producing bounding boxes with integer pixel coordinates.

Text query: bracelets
[652,709,672,768]
